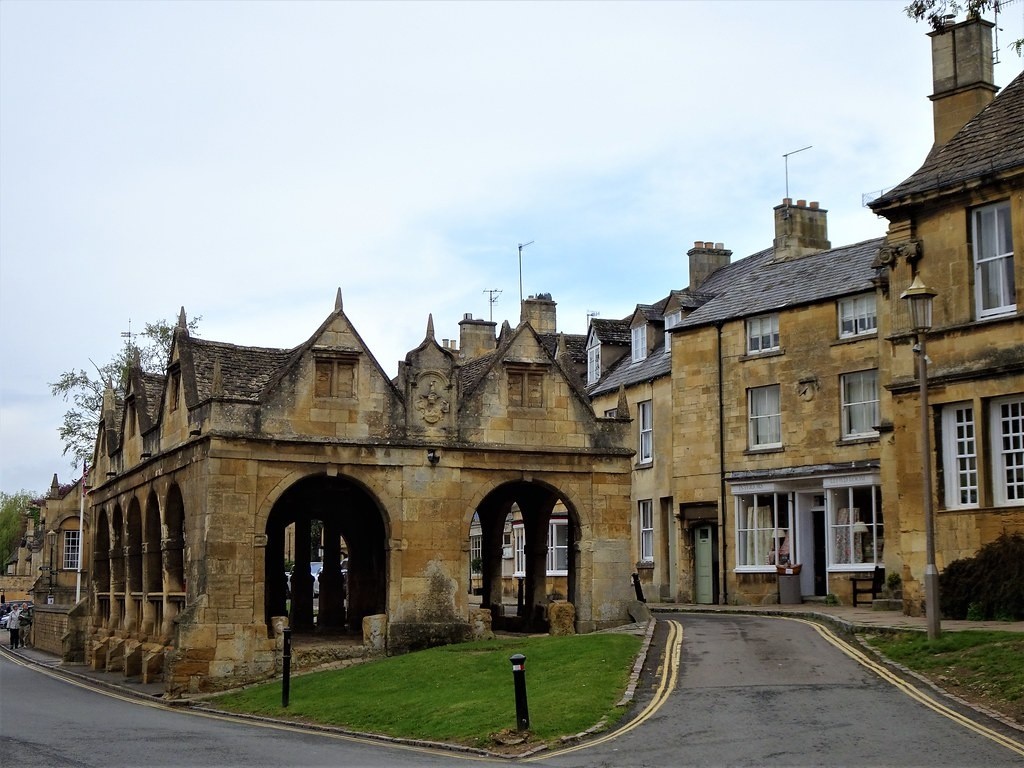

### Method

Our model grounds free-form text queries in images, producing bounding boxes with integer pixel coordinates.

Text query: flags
[81,463,88,497]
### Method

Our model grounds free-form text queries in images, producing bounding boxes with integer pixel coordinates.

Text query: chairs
[848,565,883,607]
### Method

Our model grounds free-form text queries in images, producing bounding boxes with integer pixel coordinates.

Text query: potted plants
[470,557,484,596]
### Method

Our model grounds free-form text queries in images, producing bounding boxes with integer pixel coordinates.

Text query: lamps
[427,448,440,464]
[850,522,869,545]
[46,529,58,549]
[20,534,34,547]
[771,526,785,548]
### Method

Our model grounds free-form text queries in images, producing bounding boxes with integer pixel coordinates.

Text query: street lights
[898,270,941,642]
[39,530,59,604]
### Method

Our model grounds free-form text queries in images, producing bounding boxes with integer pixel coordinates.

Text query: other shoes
[24,646,27,648]
[10,647,13,650]
[18,645,22,648]
[15,646,18,648]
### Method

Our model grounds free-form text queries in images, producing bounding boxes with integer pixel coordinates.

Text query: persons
[6,603,11,613]
[7,604,22,650]
[0,603,5,621]
[17,603,33,647]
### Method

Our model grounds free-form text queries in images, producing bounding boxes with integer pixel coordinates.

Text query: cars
[339,558,348,598]
[285,562,324,598]
[0,600,35,631]
